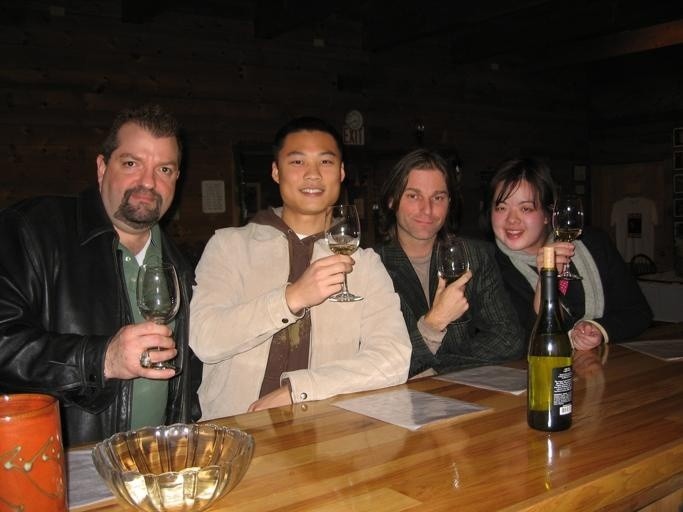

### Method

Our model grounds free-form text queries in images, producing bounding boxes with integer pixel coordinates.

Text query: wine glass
[434,238,473,326]
[136,262,180,370]
[551,195,586,282]
[323,204,364,303]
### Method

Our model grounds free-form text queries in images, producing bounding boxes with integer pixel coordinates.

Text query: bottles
[526,246,573,431]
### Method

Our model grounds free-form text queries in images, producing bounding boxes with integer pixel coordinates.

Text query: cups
[0,393,69,512]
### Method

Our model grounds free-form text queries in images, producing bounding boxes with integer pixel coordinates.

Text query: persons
[369,148,527,381]
[183,114,414,424]
[0,101,203,448]
[478,152,654,354]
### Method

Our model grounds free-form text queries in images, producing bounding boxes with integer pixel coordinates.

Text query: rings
[141,352,155,370]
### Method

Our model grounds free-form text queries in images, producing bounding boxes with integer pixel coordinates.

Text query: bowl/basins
[91,422,254,512]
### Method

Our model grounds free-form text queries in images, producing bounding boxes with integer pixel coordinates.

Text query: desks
[58,318,682,512]
